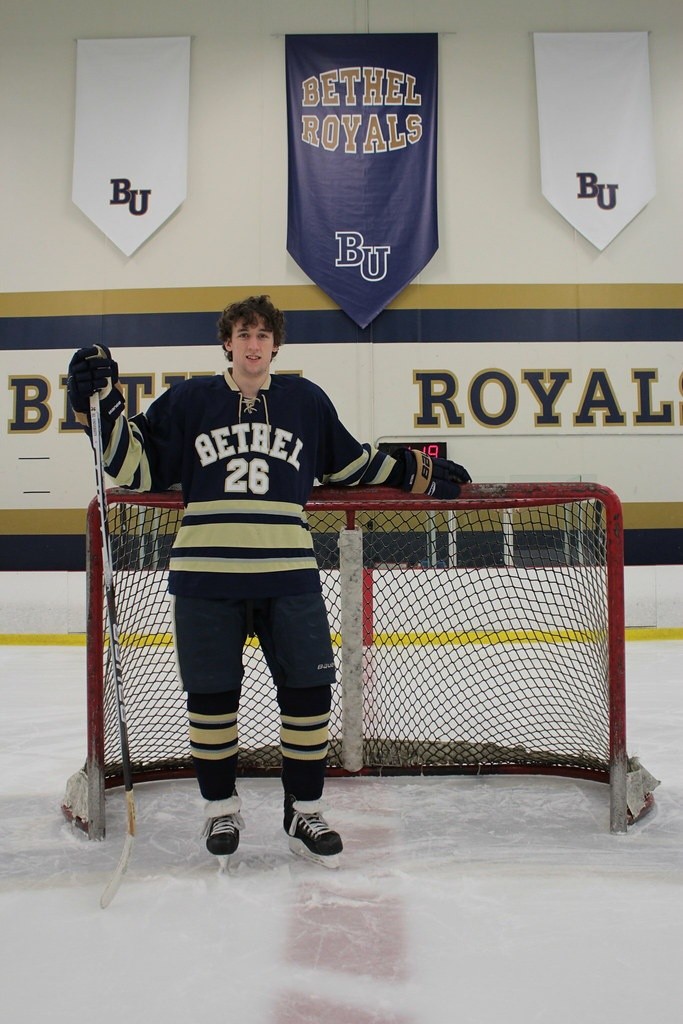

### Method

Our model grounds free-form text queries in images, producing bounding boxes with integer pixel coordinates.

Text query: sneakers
[199,789,246,874]
[284,794,344,869]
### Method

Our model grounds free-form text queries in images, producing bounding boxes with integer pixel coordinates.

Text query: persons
[69,293,471,871]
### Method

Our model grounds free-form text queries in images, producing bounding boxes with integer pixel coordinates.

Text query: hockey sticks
[90,387,137,909]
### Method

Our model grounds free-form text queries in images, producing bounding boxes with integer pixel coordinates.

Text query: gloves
[67,343,126,428]
[392,447,473,500]
